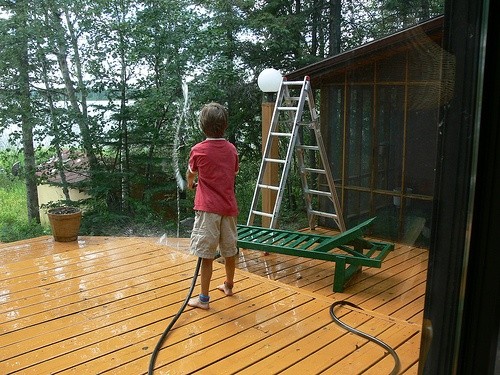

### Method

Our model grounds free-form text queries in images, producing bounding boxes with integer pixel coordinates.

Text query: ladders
[245,74,347,256]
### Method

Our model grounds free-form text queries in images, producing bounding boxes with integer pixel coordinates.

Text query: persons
[186,103,239,310]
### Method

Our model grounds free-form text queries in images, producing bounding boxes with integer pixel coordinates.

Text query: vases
[47,207,82,242]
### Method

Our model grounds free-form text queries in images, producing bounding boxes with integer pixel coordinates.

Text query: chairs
[213,225,396,292]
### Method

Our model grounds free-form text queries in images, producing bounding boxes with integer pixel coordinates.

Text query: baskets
[47,207,82,242]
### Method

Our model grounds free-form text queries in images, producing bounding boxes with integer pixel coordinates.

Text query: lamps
[258,69,283,102]
